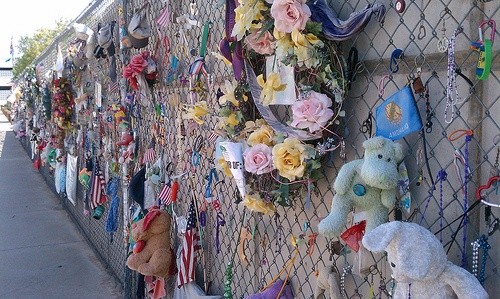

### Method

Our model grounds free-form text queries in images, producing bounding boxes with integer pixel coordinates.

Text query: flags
[158,2,170,30]
[107,74,119,95]
[90,158,108,210]
[375,82,426,140]
[139,138,158,164]
[176,193,205,289]
[157,180,174,206]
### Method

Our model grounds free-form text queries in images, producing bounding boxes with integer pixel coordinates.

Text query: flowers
[47,77,76,130]
[177,0,347,216]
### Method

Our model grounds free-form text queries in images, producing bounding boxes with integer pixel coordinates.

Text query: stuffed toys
[245,263,343,299]
[363,220,490,299]
[72,21,96,58]
[126,207,175,278]
[317,136,407,238]
